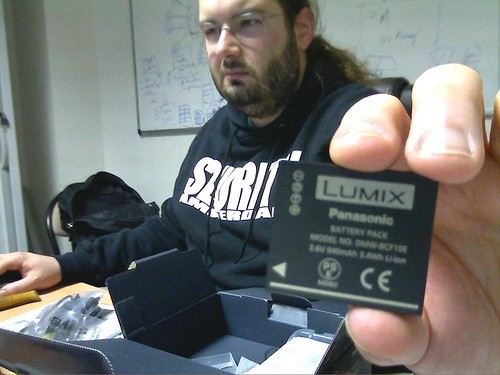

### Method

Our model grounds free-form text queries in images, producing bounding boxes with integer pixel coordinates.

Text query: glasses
[197,12,287,41]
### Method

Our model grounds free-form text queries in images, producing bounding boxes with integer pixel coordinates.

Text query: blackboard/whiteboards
[127,1,499,138]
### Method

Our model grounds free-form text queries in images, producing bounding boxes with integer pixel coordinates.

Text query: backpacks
[60,171,162,254]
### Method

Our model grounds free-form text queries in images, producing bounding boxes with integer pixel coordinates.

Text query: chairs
[46,190,80,257]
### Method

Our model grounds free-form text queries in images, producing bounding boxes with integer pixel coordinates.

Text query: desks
[0,282,119,375]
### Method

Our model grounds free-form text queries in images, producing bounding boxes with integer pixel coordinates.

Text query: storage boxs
[1,246,368,375]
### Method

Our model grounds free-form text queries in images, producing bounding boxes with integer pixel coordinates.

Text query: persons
[0,0,500,375]
